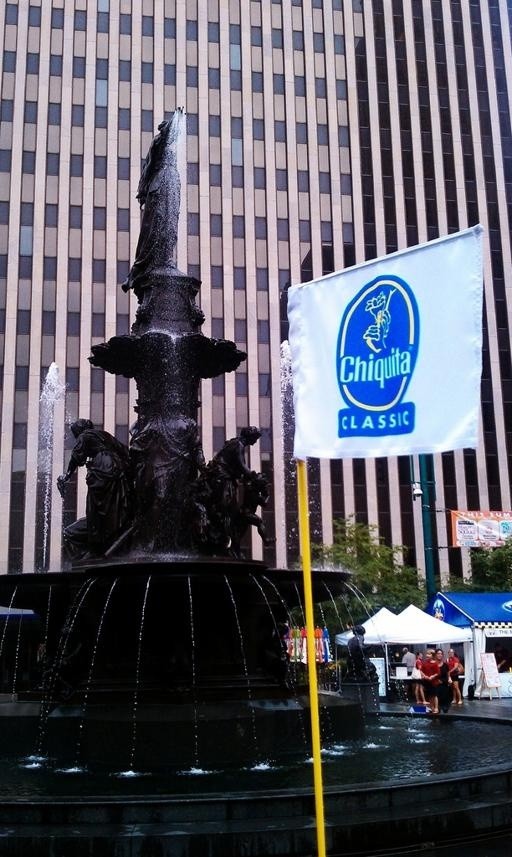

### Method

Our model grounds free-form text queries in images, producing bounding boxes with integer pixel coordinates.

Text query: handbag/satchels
[412,667,421,680]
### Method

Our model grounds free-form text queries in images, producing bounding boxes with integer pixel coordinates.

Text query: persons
[495,642,512,674]
[401,646,464,716]
[346,625,385,682]
[198,426,262,559]
[56,419,130,563]
[232,471,276,548]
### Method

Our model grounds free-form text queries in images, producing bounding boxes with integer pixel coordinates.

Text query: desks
[390,675,465,703]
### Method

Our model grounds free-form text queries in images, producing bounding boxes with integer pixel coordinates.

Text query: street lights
[408,479,440,610]
